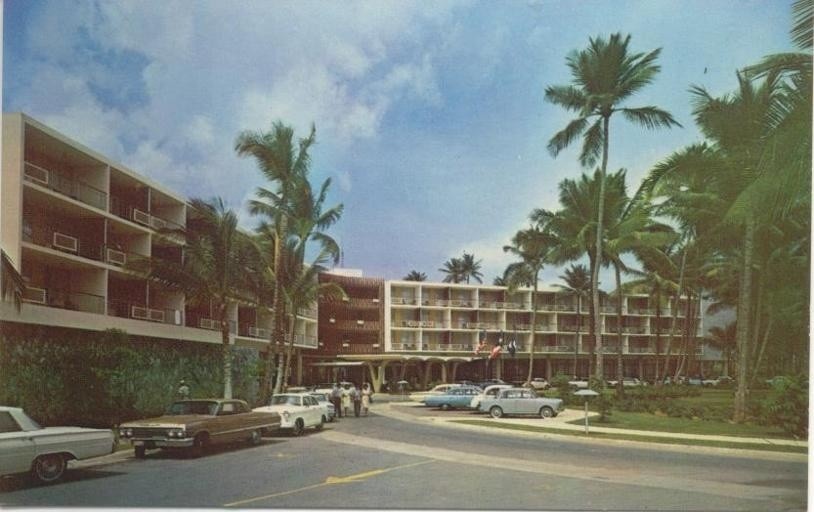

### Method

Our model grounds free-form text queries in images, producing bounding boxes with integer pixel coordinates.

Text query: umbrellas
[398,380,409,383]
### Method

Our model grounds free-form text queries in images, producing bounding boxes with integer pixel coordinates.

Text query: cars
[120,396,281,456]
[524,377,551,389]
[0,405,117,481]
[472,385,564,420]
[607,374,732,388]
[288,381,357,415]
[568,375,589,389]
[253,391,325,437]
[422,380,483,410]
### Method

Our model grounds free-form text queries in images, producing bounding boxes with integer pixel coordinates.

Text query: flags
[489,331,504,360]
[475,330,488,355]
[508,332,516,357]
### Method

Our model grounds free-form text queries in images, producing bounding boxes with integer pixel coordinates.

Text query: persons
[179,379,189,398]
[332,381,373,417]
[401,383,405,394]
